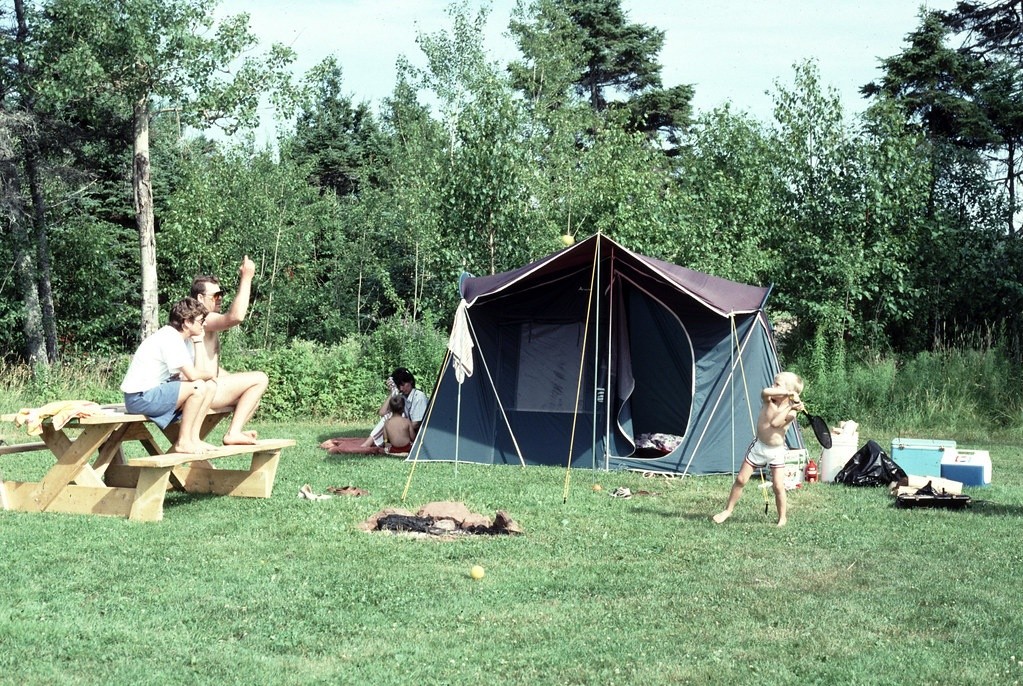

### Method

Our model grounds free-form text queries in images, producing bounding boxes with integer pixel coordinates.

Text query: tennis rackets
[795,399,833,449]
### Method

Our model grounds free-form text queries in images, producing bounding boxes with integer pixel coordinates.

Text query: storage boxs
[940,447,993,487]
[891,438,957,479]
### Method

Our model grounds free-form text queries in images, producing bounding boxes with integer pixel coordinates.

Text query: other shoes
[298,483,331,501]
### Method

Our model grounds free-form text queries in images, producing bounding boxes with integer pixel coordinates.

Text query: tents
[406,229,808,476]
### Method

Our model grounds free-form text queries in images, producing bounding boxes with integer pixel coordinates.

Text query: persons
[120,297,221,454]
[361,367,428,448]
[383,397,415,453]
[191,255,269,445]
[713,372,803,525]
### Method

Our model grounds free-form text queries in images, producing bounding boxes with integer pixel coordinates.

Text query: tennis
[593,484,601,491]
[470,565,484,578]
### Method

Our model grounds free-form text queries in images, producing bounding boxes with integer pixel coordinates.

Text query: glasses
[202,291,223,301]
[197,318,205,325]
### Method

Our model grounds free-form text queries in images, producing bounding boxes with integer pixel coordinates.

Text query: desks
[1,400,266,518]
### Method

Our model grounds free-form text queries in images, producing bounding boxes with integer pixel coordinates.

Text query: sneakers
[607,486,632,500]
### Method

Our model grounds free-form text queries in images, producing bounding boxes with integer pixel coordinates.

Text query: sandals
[327,485,371,497]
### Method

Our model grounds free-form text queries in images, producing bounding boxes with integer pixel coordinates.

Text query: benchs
[0,436,297,523]
